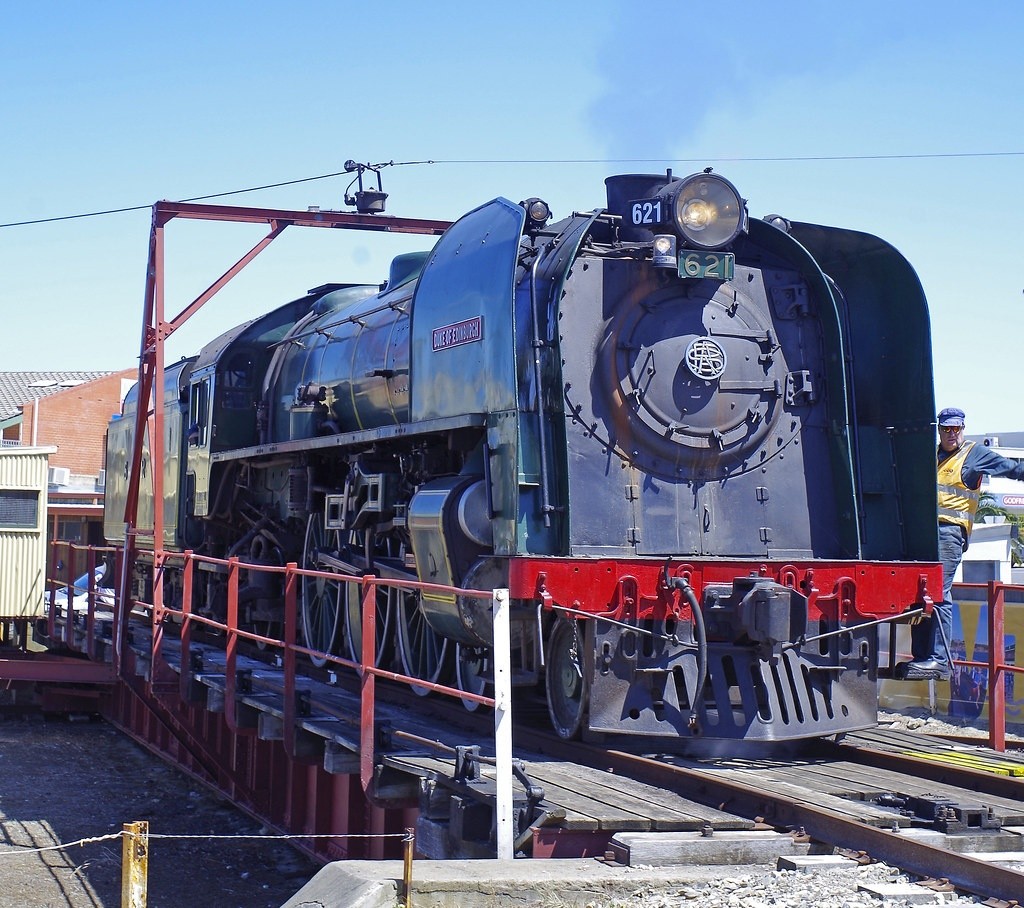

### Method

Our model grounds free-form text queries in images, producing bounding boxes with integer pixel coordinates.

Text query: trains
[101,171,953,763]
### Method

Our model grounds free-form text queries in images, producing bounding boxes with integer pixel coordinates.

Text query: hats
[937,408,966,427]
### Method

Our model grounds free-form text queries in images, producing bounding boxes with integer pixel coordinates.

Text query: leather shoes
[895,660,951,682]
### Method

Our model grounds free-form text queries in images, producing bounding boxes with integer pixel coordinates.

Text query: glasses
[939,425,963,433]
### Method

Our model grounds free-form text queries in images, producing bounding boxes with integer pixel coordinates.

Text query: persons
[896,408,1024,681]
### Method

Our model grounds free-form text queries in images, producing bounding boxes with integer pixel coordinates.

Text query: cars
[45,563,152,620]
